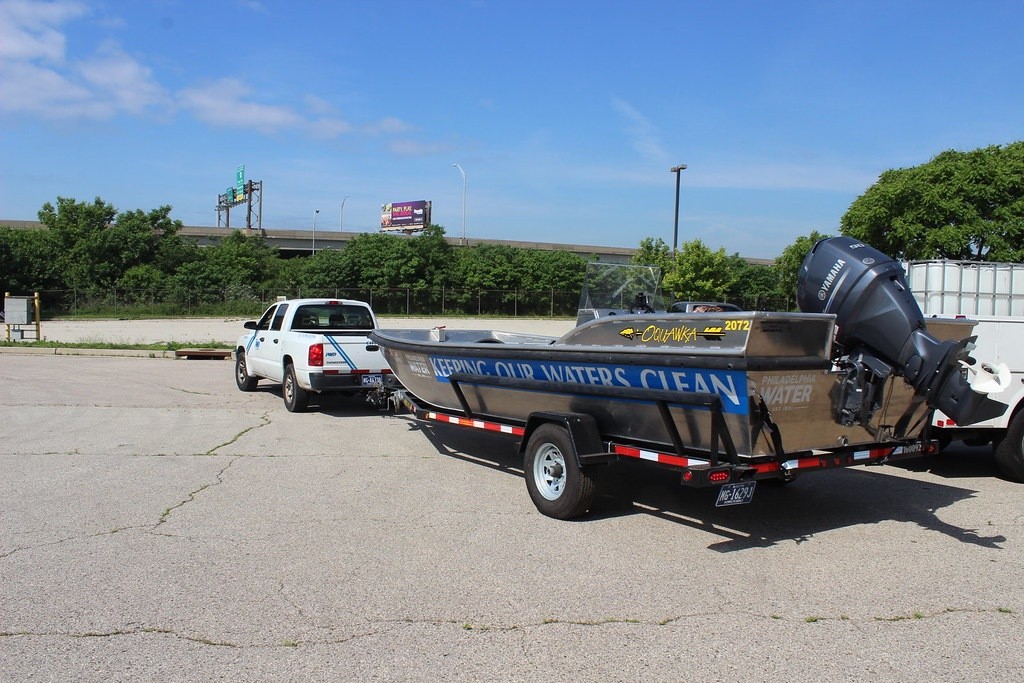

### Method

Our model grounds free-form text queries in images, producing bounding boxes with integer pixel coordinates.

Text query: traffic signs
[235,165,245,202]
[225,186,235,204]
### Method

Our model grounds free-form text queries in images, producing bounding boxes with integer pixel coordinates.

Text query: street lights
[311,208,321,256]
[453,162,466,238]
[670,164,689,258]
[340,194,349,233]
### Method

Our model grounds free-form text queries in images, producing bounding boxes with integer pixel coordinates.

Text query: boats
[365,235,1013,467]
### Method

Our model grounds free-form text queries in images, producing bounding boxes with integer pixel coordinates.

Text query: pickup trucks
[234,297,398,414]
